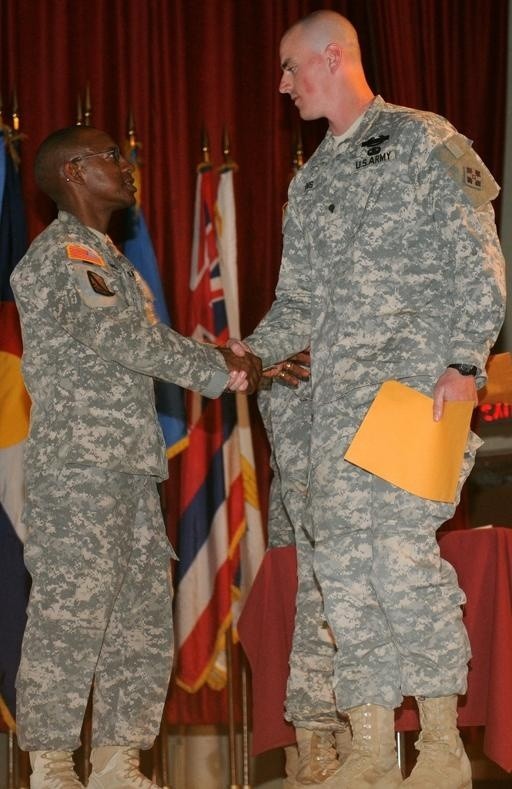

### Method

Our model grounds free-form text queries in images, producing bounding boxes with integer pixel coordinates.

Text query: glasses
[64,145,121,183]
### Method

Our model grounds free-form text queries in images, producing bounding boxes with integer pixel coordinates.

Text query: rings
[279,372,287,378]
[286,361,293,369]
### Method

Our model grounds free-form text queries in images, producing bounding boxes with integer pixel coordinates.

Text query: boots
[402,693,475,789]
[284,699,402,789]
[28,750,86,789]
[87,744,162,789]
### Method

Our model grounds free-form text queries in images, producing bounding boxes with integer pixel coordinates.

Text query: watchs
[448,364,477,377]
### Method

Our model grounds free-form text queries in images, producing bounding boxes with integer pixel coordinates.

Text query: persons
[10,125,312,789]
[256,351,354,788]
[225,9,508,789]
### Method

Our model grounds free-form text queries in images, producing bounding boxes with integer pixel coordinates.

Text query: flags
[0,124,33,734]
[110,149,193,462]
[171,163,246,694]
[216,168,264,694]
